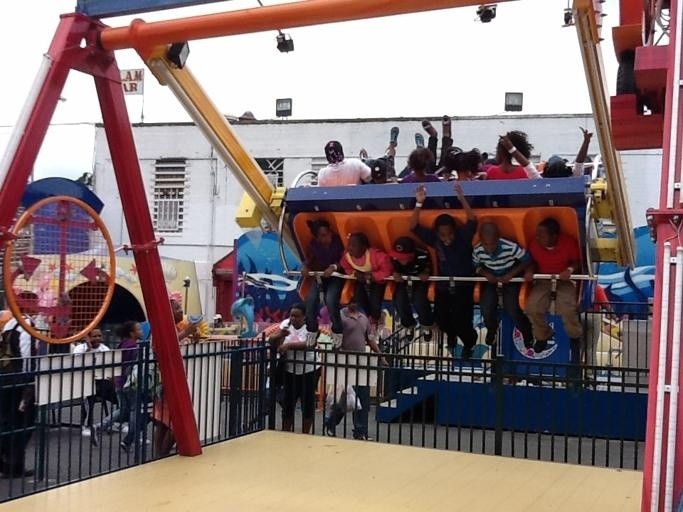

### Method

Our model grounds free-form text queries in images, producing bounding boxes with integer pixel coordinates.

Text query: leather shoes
[446,334,457,347]
[463,333,477,352]
[423,330,431,342]
[2,470,33,479]
[405,328,414,343]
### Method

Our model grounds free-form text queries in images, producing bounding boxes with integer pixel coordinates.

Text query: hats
[325,141,343,153]
[370,157,388,180]
[393,236,420,256]
[212,313,223,319]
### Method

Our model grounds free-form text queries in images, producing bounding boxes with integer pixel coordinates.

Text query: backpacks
[0,318,29,374]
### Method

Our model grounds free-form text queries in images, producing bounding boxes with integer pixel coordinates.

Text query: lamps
[167,42,189,67]
[505,92,524,114]
[275,98,292,120]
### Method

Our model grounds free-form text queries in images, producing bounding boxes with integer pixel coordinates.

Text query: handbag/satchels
[271,350,291,411]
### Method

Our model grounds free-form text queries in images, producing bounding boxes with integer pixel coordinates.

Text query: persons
[300,183,593,357]
[254,295,390,440]
[317,115,593,186]
[0,302,226,480]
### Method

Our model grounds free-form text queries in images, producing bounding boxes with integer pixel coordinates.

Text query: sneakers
[421,120,436,136]
[120,442,133,454]
[376,311,386,330]
[331,331,343,349]
[353,429,374,441]
[485,331,496,346]
[569,334,584,352]
[359,149,368,162]
[390,127,399,146]
[534,330,556,353]
[441,115,451,138]
[304,331,318,348]
[523,335,532,349]
[414,133,424,148]
[92,423,101,447]
[324,416,335,437]
